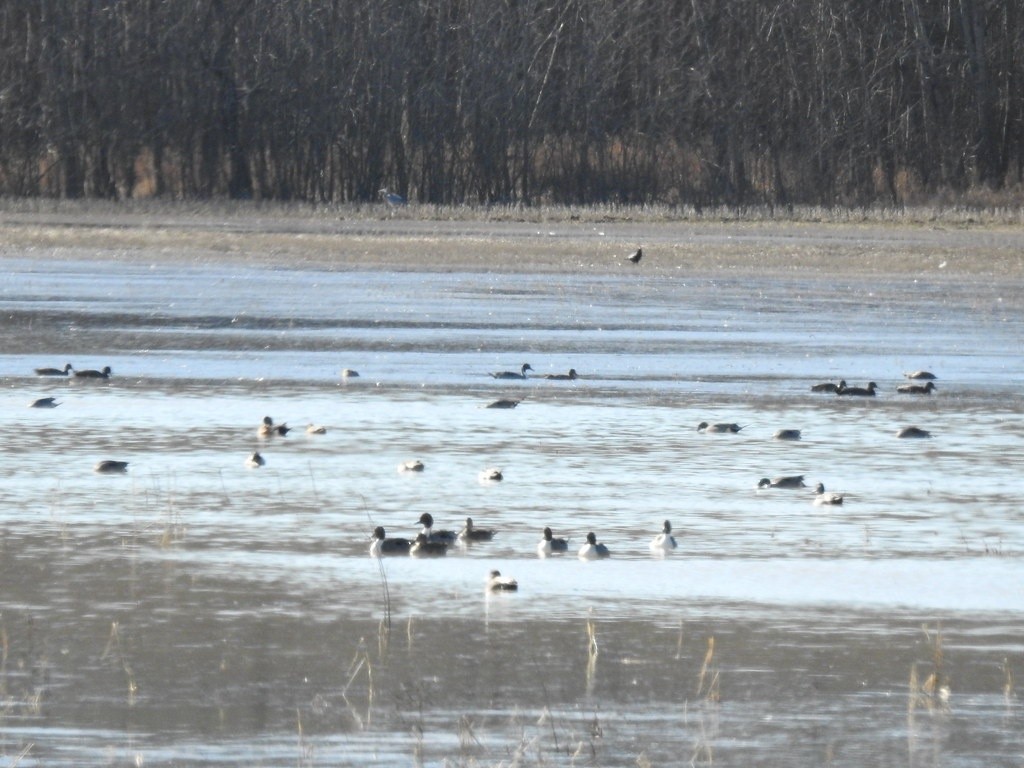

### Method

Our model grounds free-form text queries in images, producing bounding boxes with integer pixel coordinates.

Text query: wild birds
[896,424,934,440]
[34,361,113,378]
[489,361,537,380]
[812,483,844,507]
[537,526,571,559]
[895,367,939,394]
[758,476,807,488]
[343,367,357,381]
[487,399,518,409]
[697,421,751,433]
[648,521,678,553]
[304,422,323,431]
[478,466,506,485]
[257,416,291,441]
[489,567,518,592]
[249,452,267,468]
[29,396,60,407]
[545,368,580,381]
[578,530,609,558]
[92,460,130,473]
[810,375,881,400]
[772,429,804,442]
[372,513,496,557]
[625,244,643,263]
[397,457,424,472]
